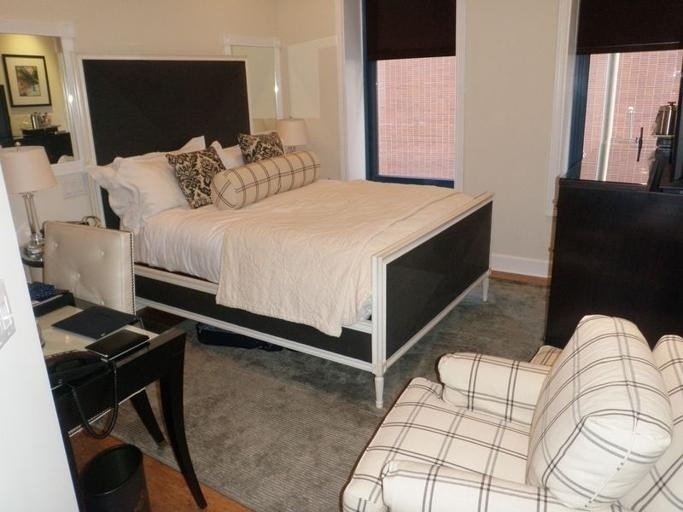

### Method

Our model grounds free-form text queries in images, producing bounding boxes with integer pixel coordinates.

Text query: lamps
[2,146,58,264]
[277,119,309,150]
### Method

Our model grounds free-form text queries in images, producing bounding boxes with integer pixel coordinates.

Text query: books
[86,329,149,360]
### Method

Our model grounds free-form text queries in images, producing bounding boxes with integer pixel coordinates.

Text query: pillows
[209,150,321,209]
[235,133,284,165]
[166,149,227,211]
[207,140,222,150]
[88,135,206,221]
[528,313,672,508]
[211,138,244,168]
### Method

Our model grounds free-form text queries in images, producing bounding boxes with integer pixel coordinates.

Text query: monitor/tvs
[646,149,667,188]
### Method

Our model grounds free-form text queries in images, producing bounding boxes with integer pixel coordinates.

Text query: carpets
[88,274,550,512]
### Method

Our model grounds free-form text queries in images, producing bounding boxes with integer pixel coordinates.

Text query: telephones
[43,350,111,384]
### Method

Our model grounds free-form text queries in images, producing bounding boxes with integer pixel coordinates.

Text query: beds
[77,52,495,409]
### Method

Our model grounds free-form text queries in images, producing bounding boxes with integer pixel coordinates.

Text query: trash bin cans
[78,444,152,512]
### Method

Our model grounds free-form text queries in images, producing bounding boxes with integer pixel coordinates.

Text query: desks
[24,280,207,512]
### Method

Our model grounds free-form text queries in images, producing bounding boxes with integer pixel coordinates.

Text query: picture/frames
[2,53,52,108]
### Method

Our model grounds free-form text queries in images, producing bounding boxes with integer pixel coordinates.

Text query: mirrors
[0,35,80,153]
[224,38,284,133]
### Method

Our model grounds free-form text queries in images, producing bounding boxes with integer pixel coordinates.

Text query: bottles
[663,101,676,135]
[31,115,37,130]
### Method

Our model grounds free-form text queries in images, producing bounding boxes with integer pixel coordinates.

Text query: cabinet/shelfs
[542,177,683,349]
[17,128,73,163]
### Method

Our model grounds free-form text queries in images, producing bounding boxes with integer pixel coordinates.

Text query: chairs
[340,314,682,512]
[44,220,167,446]
